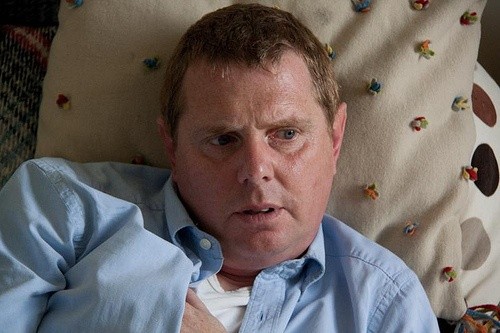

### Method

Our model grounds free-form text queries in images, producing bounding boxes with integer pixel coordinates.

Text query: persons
[0,2,444,333]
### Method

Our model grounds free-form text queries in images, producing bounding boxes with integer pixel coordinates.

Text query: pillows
[36,0,488,320]
